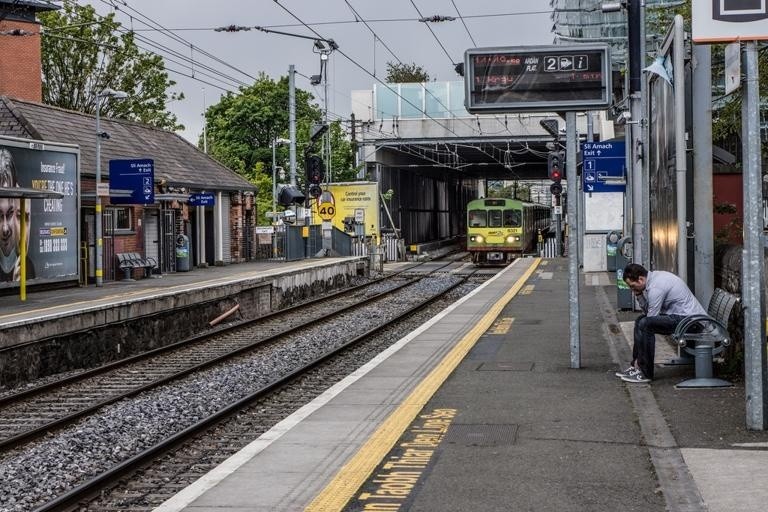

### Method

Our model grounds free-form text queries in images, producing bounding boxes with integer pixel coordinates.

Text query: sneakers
[620,368,652,383]
[615,366,635,378]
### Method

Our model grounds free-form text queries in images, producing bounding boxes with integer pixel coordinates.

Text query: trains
[465,196,551,267]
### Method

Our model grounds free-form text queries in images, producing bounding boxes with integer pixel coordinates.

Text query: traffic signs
[318,202,336,220]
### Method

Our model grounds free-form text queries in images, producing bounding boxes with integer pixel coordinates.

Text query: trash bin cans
[175,234,190,272]
[606,231,633,311]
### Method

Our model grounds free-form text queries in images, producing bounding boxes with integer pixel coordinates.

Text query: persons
[0,196,34,283]
[14,198,31,257]
[616,263,709,383]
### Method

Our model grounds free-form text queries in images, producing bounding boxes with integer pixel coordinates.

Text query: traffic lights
[303,125,328,199]
[537,119,565,195]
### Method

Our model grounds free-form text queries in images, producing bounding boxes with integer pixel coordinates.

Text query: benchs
[663,286,741,389]
[116,253,156,283]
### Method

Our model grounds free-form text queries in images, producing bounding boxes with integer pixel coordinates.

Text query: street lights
[272,138,292,259]
[93,89,128,287]
